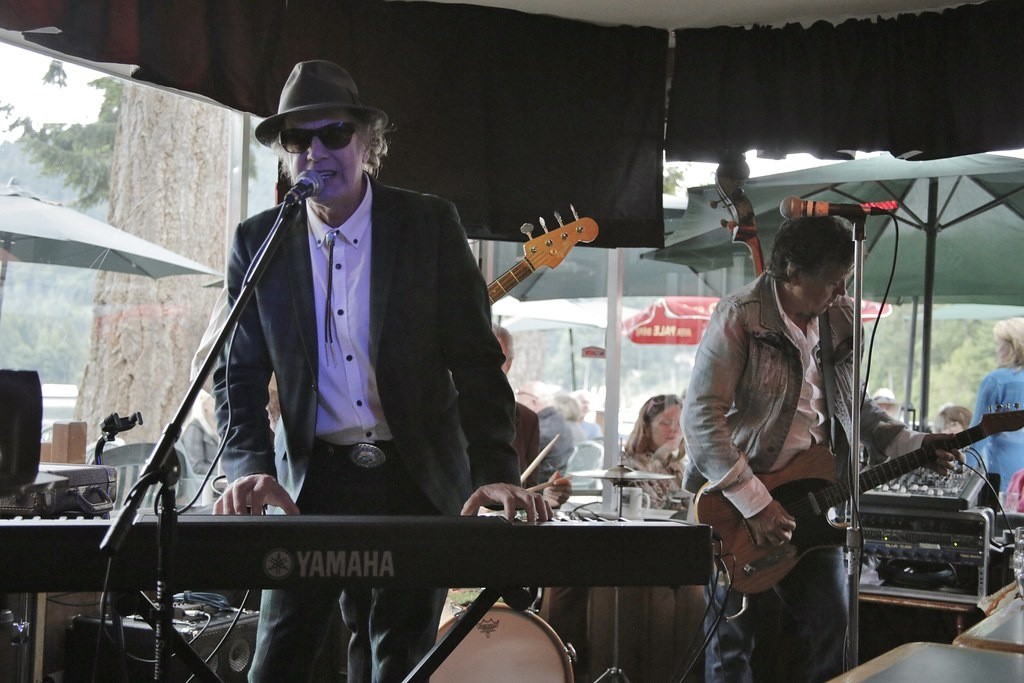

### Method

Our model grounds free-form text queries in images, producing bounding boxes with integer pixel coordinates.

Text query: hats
[874,388,896,408]
[255,59,387,148]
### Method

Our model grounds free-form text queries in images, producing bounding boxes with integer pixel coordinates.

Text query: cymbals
[568,464,677,480]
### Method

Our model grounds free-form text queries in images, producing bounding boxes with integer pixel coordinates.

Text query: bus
[466,195,758,437]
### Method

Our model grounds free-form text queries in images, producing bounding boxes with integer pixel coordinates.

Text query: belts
[312,437,401,470]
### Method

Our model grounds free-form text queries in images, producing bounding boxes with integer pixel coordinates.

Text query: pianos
[0,507,718,683]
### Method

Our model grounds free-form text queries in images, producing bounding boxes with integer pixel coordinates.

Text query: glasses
[278,122,361,154]
[647,395,677,415]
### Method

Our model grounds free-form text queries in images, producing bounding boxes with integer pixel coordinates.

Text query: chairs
[91,443,186,513]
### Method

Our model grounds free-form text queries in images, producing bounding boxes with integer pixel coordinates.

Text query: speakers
[76,606,263,683]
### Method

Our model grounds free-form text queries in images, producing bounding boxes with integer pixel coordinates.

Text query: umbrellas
[493,295,639,389]
[0,176,225,320]
[641,150,1024,431]
[626,296,893,345]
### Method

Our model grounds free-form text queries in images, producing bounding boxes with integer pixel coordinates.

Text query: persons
[967,317,1024,492]
[679,215,965,683]
[212,61,553,683]
[621,395,687,487]
[935,406,971,434]
[491,324,605,508]
[872,387,898,416]
[182,390,221,477]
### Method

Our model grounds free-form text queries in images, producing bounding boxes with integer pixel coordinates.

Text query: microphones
[779,194,890,219]
[283,170,326,206]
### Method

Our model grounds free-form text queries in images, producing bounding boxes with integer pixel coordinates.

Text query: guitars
[692,400,1024,597]
[486,203,601,308]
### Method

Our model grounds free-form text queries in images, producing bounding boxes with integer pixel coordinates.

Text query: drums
[429,596,575,683]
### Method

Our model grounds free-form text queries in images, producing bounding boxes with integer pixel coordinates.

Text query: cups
[616,487,650,522]
[1013,526,1024,603]
